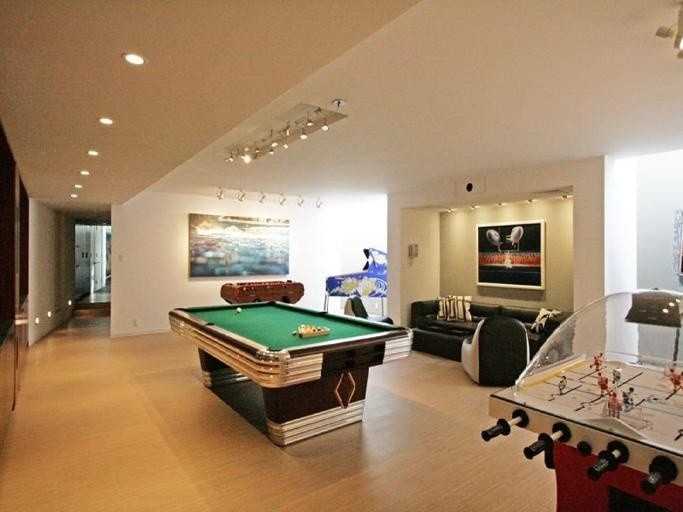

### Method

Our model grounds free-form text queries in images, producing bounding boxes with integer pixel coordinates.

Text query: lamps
[224,100,349,164]
[656,0,683,59]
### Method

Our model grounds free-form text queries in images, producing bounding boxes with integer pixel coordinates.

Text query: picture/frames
[476,220,546,291]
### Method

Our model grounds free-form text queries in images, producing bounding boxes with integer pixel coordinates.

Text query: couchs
[411,297,574,361]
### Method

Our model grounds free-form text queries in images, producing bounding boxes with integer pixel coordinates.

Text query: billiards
[292,331,296,335]
[299,323,329,337]
[236,307,242,312]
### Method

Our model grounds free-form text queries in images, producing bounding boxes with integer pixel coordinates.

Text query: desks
[169,301,414,447]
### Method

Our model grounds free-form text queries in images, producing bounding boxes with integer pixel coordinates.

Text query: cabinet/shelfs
[0,119,29,448]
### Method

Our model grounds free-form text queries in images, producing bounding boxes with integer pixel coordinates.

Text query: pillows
[437,296,446,321]
[530,308,562,333]
[446,294,472,322]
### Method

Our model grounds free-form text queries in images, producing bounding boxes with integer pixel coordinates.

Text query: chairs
[344,296,394,325]
[461,316,530,387]
